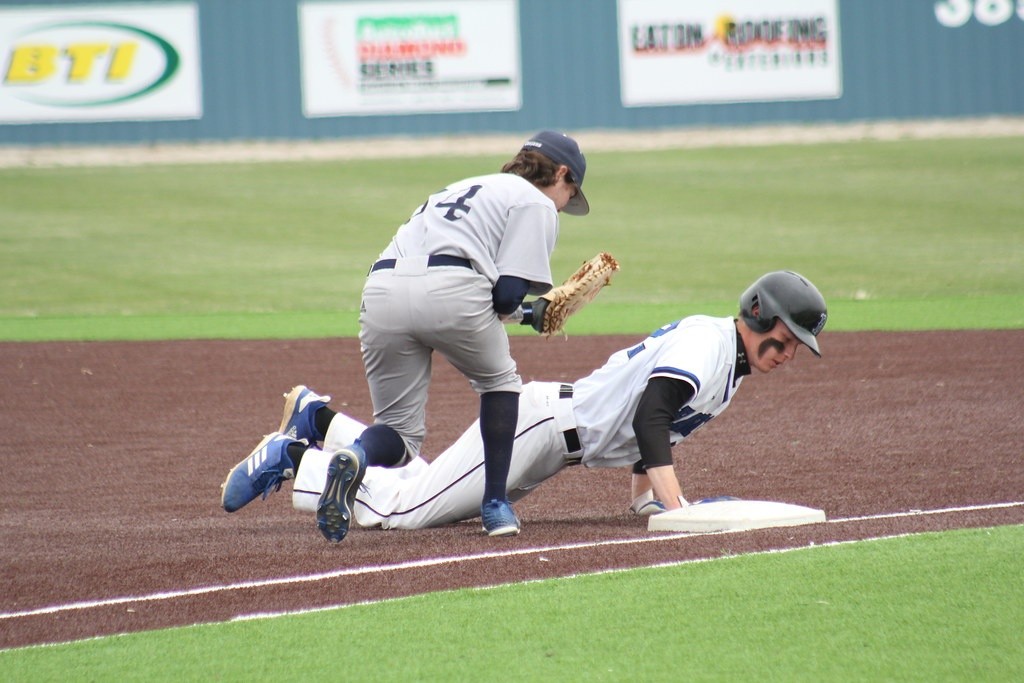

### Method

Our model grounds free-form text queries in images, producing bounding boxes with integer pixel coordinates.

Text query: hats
[523,131,591,216]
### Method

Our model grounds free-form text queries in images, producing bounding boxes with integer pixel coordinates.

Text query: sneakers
[221,430,304,514]
[481,497,522,537]
[315,442,367,546]
[279,384,330,448]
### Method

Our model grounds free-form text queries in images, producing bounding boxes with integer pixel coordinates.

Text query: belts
[365,254,475,273]
[558,381,585,466]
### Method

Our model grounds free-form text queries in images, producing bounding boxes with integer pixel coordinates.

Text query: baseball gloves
[533,253,619,337]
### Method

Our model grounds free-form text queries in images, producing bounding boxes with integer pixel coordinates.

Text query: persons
[315,126,620,545]
[220,268,826,532]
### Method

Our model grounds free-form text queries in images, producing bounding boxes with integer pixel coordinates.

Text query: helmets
[739,271,826,358]
